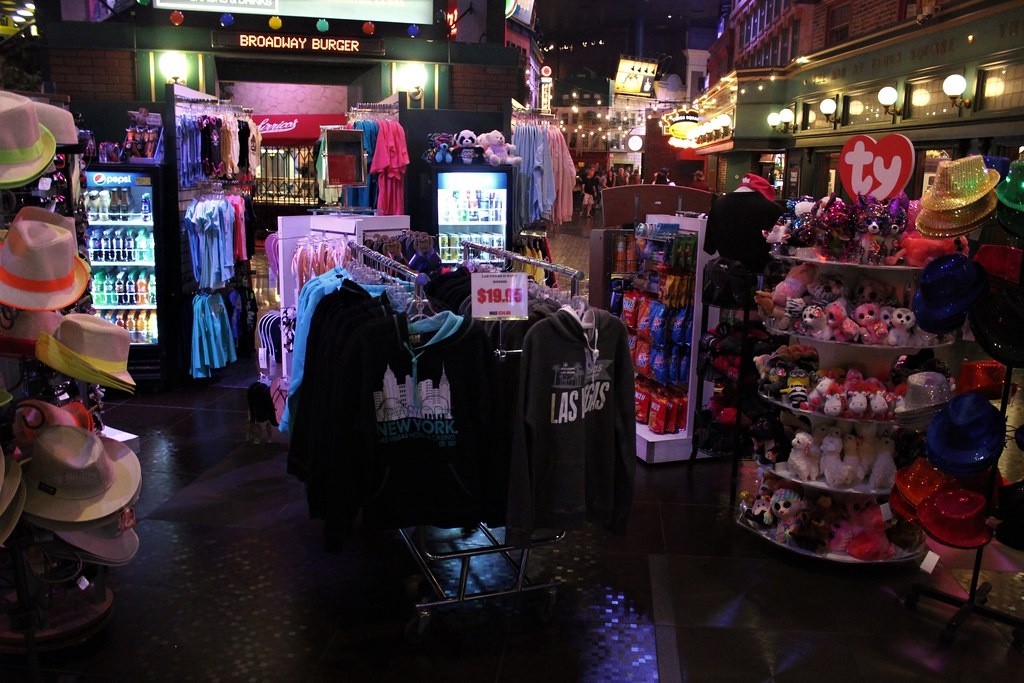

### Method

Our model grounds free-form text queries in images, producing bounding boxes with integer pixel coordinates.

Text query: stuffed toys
[754,343,957,422]
[738,473,927,561]
[749,404,928,490]
[753,265,954,349]
[421,130,523,166]
[762,192,969,268]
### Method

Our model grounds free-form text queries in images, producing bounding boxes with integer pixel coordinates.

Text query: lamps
[820,99,841,123]
[877,87,901,116]
[767,112,786,133]
[943,74,971,109]
[779,109,797,130]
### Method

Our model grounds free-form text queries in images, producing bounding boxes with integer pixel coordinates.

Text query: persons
[704,173,785,275]
[690,170,714,191]
[572,160,676,219]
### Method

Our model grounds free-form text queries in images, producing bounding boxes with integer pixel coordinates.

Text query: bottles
[82,188,157,344]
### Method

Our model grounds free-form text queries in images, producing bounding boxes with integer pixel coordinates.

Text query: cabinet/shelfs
[736,233,957,562]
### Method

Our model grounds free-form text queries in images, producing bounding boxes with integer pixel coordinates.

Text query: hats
[0,399,143,565]
[912,155,1024,369]
[890,360,1020,549]
[659,167,671,173]
[0,206,136,395]
[691,170,706,181]
[738,173,775,203]
[0,90,79,189]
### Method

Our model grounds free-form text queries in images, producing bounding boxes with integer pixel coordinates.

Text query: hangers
[514,108,560,131]
[195,178,242,204]
[294,227,594,327]
[347,102,397,123]
[179,96,254,123]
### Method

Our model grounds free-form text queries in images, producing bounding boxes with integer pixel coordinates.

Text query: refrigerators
[408,163,513,276]
[80,164,163,390]
